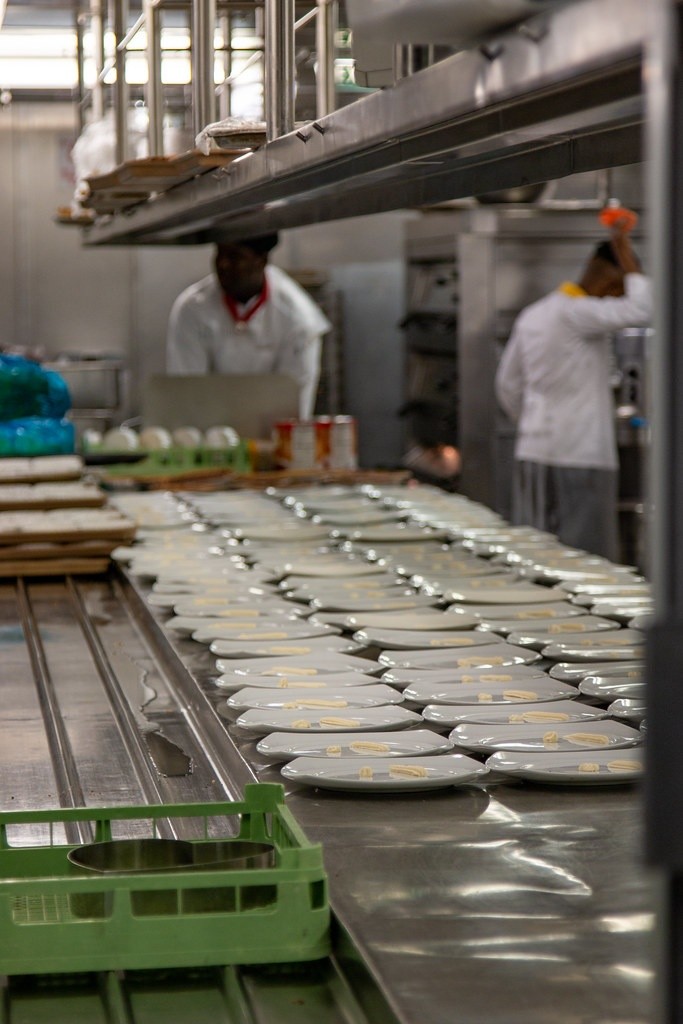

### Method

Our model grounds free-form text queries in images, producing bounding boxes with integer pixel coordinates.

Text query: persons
[166,231,333,474]
[492,207,651,566]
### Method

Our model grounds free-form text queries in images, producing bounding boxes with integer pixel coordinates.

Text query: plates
[109,484,653,791]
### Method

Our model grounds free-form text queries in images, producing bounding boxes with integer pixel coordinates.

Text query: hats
[595,240,642,273]
[208,204,278,254]
